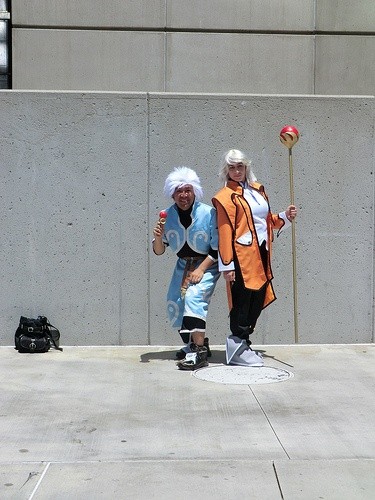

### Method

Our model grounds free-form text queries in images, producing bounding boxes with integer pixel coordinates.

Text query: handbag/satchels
[14,315,63,353]
[17,335,50,353]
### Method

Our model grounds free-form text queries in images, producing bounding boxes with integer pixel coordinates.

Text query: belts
[180,256,207,262]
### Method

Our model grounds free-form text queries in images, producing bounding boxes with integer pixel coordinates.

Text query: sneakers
[175,337,212,371]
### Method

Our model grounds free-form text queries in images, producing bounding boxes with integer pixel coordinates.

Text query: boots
[225,335,265,367]
